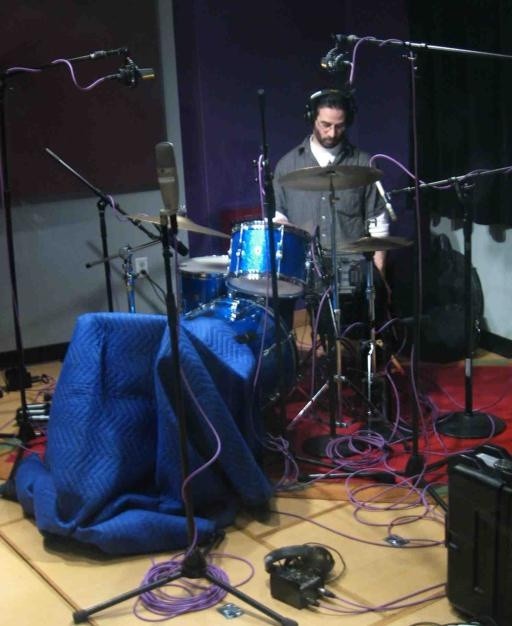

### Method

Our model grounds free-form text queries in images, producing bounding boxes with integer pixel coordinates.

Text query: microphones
[319,56,351,72]
[155,141,180,234]
[374,179,398,223]
[118,65,155,88]
[151,224,190,256]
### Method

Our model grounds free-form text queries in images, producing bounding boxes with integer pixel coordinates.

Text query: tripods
[297,49,460,512]
[0,77,47,480]
[285,204,410,453]
[72,225,299,626]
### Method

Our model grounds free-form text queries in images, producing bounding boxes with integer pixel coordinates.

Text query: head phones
[263,544,336,574]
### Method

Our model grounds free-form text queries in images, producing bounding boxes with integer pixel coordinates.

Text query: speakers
[406,0,512,230]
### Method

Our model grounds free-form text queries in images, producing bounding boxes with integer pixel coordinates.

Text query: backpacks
[392,233,483,362]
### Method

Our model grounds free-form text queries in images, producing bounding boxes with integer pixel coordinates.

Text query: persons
[270,80,392,409]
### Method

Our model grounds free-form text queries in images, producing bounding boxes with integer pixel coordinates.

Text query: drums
[224,218,313,299]
[184,291,304,416]
[177,254,232,314]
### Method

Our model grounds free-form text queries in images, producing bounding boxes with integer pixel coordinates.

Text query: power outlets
[136,257,147,279]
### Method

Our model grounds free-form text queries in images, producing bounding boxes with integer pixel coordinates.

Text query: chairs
[279,306,403,463]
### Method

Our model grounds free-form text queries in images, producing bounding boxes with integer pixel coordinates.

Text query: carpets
[0,362,512,507]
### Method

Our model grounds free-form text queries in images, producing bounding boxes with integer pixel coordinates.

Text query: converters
[270,568,323,610]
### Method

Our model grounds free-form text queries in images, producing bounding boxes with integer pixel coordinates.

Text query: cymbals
[277,165,385,191]
[320,237,415,255]
[122,213,231,240]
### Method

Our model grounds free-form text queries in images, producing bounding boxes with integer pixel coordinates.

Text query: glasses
[314,119,347,133]
